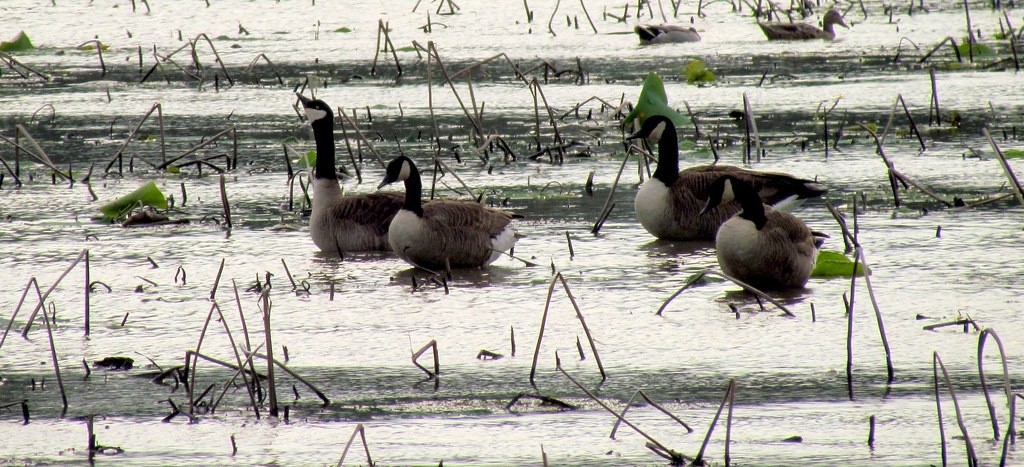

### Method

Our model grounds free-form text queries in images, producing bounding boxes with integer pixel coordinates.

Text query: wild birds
[377,154,527,274]
[757,9,850,41]
[633,16,701,46]
[294,91,431,253]
[627,114,828,241]
[699,175,831,291]
